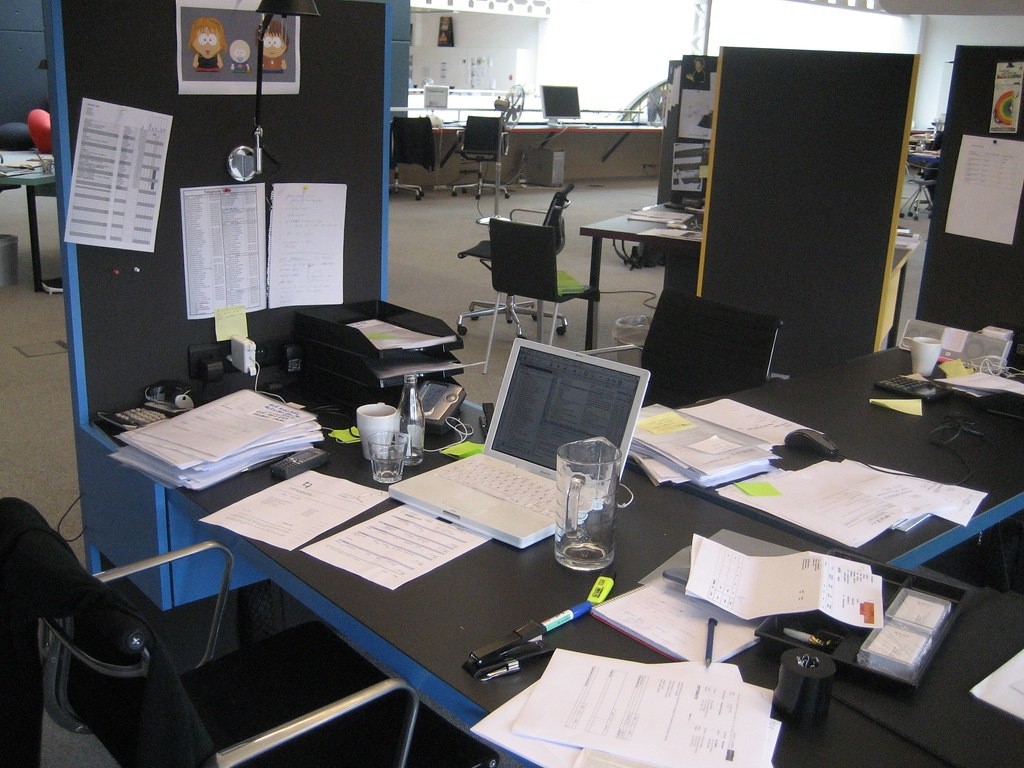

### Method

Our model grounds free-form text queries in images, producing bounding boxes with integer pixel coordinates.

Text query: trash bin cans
[0,233,19,286]
[613,314,653,368]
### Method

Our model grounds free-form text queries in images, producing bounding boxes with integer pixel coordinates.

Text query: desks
[579,201,701,351]
[431,124,664,133]
[102,347,1024,768]
[0,149,55,293]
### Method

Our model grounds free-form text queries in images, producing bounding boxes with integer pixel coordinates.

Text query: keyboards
[550,123,591,129]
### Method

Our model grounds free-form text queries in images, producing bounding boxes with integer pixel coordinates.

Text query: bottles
[916,140,922,151]
[394,375,425,466]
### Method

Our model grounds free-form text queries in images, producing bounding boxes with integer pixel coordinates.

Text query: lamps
[226,0,320,183]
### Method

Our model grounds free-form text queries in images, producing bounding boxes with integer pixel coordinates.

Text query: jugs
[555,436,622,571]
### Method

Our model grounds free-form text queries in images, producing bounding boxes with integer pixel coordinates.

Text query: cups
[902,337,942,376]
[356,402,400,460]
[652,122,662,127]
[40,159,52,173]
[647,122,650,125]
[368,432,408,484]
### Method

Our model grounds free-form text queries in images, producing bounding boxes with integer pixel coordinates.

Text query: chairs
[580,290,782,408]
[390,116,435,201]
[0,495,500,768]
[455,218,591,339]
[457,183,575,271]
[899,161,937,221]
[451,115,510,199]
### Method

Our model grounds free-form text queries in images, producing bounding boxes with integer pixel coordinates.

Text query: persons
[42,278,63,293]
[924,131,944,211]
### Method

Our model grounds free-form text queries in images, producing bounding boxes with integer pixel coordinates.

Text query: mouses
[785,429,841,458]
[592,125,597,128]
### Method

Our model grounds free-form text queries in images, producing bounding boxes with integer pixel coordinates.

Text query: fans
[493,84,525,157]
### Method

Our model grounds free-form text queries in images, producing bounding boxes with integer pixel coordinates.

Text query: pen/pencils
[705,618,717,670]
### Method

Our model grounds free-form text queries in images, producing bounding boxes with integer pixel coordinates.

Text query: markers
[539,601,593,636]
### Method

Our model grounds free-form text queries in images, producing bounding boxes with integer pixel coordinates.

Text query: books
[589,582,761,663]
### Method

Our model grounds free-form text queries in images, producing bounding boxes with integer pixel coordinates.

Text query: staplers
[465,618,555,682]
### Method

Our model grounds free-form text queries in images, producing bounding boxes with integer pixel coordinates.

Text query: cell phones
[270,448,330,480]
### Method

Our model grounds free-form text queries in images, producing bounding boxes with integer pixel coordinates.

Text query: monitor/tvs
[539,85,581,126]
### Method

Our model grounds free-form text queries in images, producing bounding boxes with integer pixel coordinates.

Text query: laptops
[389,336,651,549]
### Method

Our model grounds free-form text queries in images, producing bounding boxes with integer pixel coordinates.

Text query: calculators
[96,405,171,431]
[874,375,954,402]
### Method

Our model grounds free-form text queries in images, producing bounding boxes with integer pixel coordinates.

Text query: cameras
[417,379,468,436]
